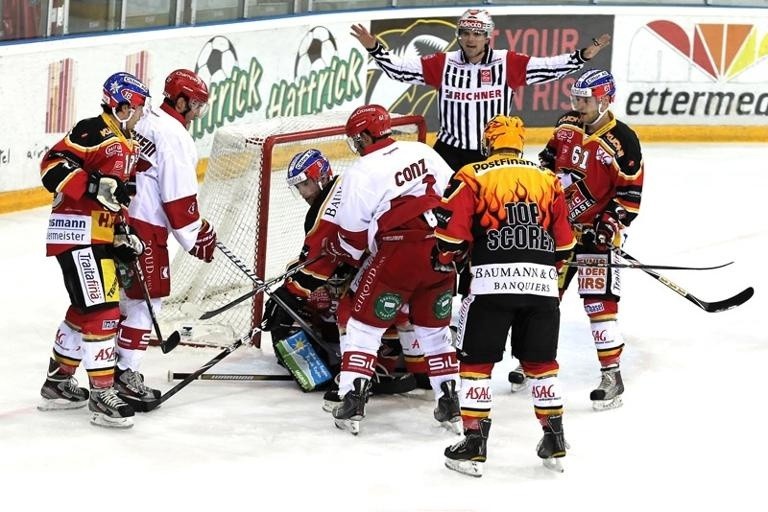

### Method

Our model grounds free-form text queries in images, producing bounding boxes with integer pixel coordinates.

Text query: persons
[506,69,644,412]
[40,72,152,418]
[261,9,611,437]
[113,68,217,402]
[431,114,578,474]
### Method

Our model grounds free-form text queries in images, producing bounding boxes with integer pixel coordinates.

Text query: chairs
[570,70,617,110]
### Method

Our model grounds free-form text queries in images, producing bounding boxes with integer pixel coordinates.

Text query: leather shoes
[84,171,135,214]
[187,219,217,264]
[112,225,145,260]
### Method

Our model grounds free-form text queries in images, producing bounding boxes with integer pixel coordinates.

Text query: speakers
[508,365,529,383]
[39,358,89,403]
[88,385,134,419]
[433,380,460,423]
[323,375,374,421]
[536,414,565,459]
[581,214,619,252]
[116,368,161,403]
[589,366,625,401]
[443,419,491,463]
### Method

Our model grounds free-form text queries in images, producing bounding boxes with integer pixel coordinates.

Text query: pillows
[102,73,152,113]
[453,9,496,41]
[163,68,210,103]
[287,148,335,189]
[343,103,392,143]
[485,115,527,151]
